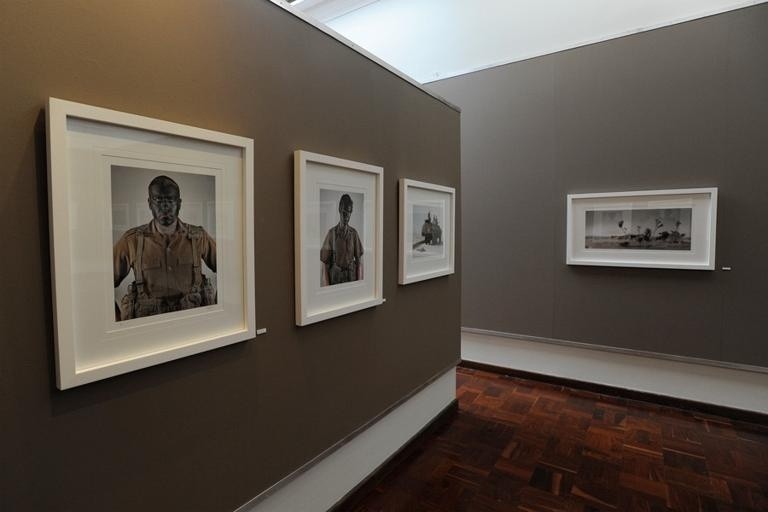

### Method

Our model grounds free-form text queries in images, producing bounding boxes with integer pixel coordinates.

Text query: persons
[320,193,364,287]
[421,219,432,245]
[113,175,216,321]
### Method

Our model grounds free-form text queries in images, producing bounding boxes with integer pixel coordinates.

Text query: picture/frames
[397,176,456,284]
[294,150,384,327]
[44,98,256,393]
[565,187,720,271]
